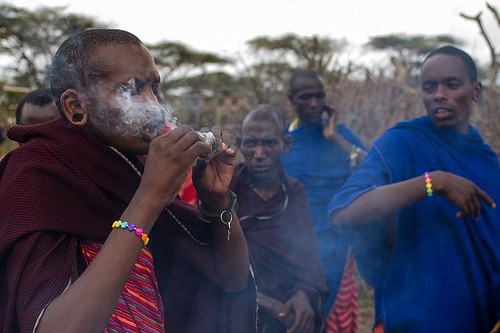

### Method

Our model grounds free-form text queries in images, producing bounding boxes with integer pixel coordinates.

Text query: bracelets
[346,144,361,168]
[111,221,149,246]
[198,187,240,241]
[424,171,432,198]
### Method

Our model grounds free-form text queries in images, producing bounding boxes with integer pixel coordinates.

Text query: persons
[259,72,369,333]
[17,86,60,147]
[0,28,250,333]
[230,105,337,333]
[328,47,500,333]
